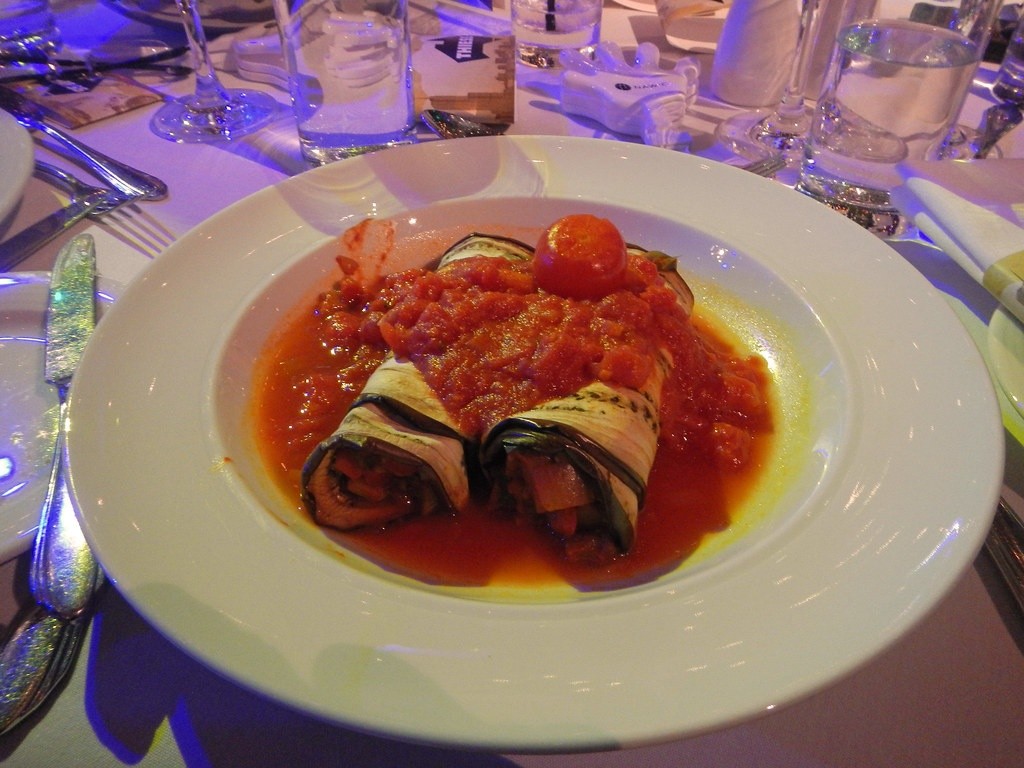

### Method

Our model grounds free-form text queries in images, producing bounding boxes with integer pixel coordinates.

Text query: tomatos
[530,213,628,303]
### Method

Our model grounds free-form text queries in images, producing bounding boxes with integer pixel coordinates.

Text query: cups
[510,1,601,69]
[0,0,64,65]
[286,0,416,167]
[800,0,1005,212]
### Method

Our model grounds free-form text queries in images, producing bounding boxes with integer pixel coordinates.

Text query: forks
[30,158,157,216]
[739,157,787,179]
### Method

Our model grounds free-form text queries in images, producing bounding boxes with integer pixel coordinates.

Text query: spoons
[0,86,168,201]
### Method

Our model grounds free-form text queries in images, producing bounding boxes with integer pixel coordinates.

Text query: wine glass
[148,0,286,144]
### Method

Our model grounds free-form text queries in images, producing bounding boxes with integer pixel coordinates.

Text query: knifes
[0,609,83,737]
[61,136,1010,759]
[1,193,106,273]
[23,234,97,611]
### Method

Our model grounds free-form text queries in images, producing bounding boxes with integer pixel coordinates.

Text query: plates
[1,270,128,567]
[1,110,35,230]
